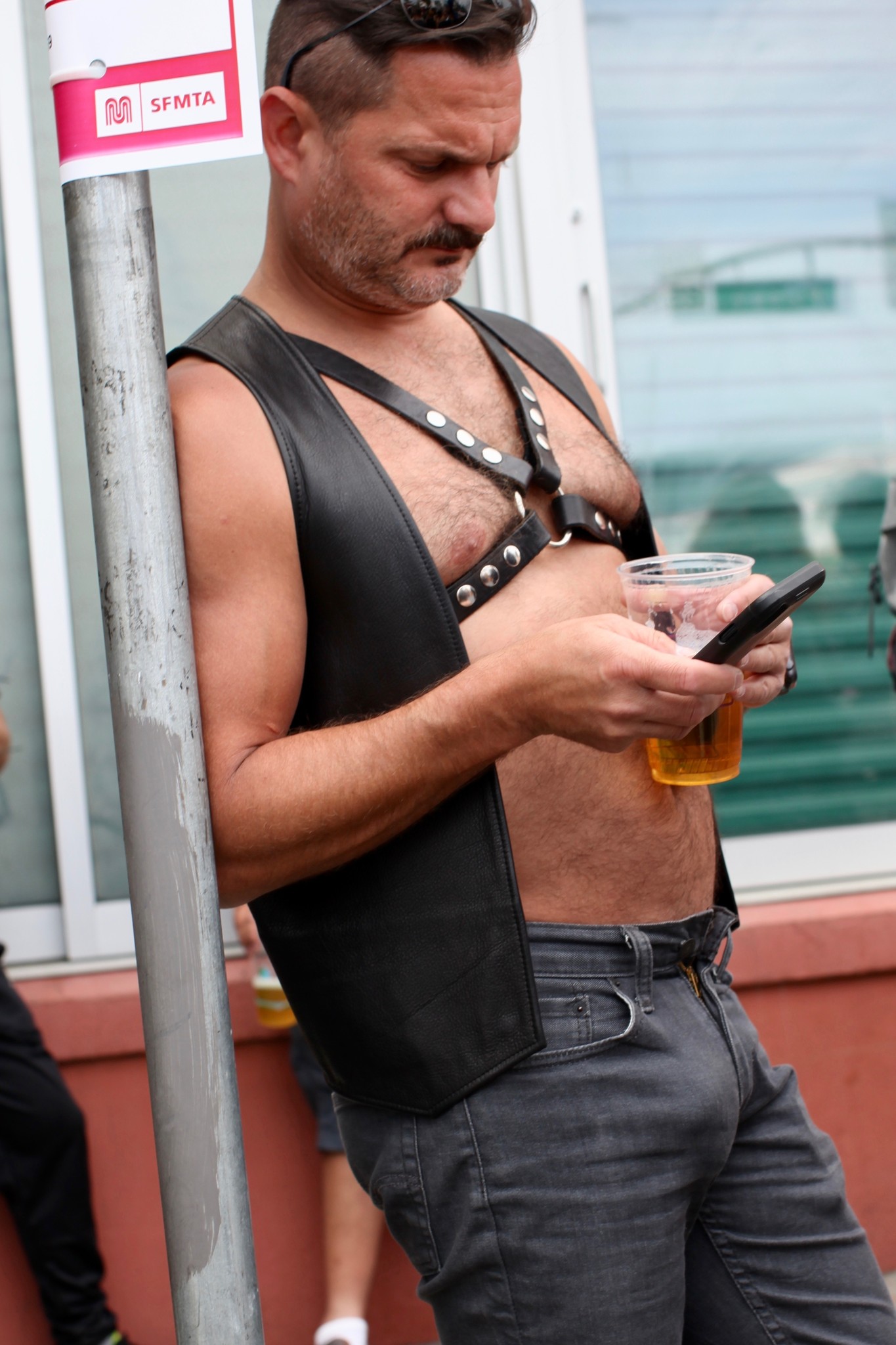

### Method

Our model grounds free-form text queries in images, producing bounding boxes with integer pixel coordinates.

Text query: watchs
[778,642,799,693]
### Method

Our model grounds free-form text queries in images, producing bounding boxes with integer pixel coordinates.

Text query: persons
[0,717,133,1344]
[159,0,895,1345]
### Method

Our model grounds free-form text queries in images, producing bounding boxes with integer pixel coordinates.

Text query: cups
[247,924,297,1031]
[618,551,755,785]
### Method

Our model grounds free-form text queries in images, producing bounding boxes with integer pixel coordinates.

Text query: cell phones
[691,561,826,665]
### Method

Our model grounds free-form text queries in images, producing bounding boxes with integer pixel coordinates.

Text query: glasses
[280,0,524,87]
